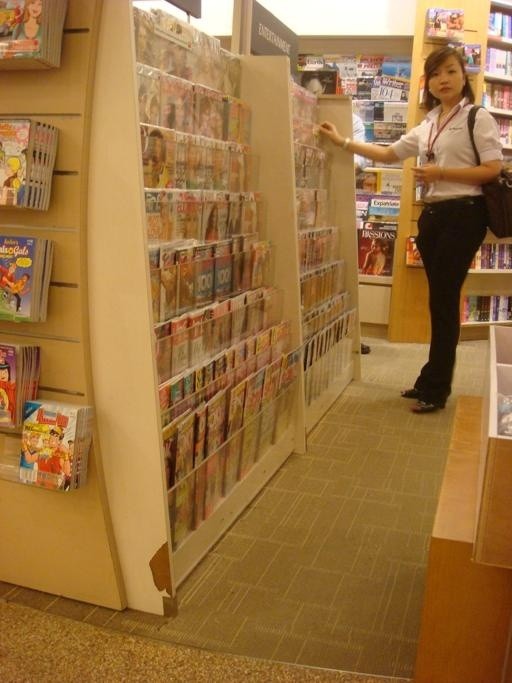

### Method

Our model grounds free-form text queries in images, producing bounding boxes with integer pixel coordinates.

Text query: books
[134,3,358,555]
[403,0,512,328]
[0,116,95,500]
[296,51,411,280]
[1,0,72,73]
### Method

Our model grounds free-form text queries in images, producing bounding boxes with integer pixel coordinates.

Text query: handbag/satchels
[483,170,512,239]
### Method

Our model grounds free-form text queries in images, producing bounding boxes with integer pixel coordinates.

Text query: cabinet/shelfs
[212,2,512,350]
[3,1,362,614]
[411,328,511,683]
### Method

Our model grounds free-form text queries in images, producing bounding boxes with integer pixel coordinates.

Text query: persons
[314,42,506,419]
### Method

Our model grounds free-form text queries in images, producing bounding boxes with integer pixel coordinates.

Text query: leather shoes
[401,387,418,398]
[410,399,442,413]
